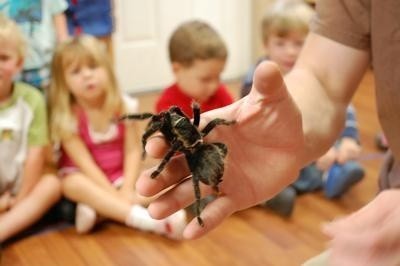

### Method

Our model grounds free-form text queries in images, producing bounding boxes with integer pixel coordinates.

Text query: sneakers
[268,185,296,219]
[154,210,187,243]
[76,203,100,234]
[323,160,364,200]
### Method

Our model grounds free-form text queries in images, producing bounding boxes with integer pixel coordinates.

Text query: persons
[136,0,400,266]
[154,18,299,218]
[0,0,69,91]
[240,0,366,200]
[48,33,187,241]
[0,15,63,244]
